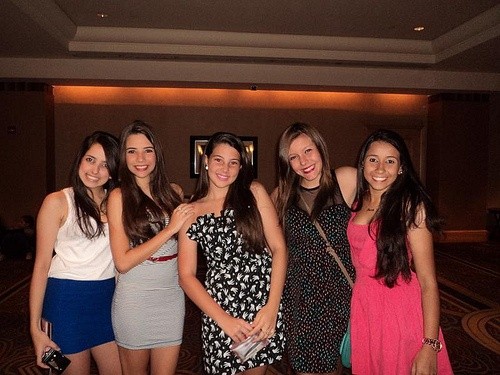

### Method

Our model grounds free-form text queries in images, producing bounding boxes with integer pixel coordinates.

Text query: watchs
[422,337,442,352]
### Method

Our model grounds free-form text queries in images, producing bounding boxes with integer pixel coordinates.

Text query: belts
[146,254,178,262]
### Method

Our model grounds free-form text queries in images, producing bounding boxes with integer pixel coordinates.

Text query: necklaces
[99,208,106,216]
[364,203,377,215]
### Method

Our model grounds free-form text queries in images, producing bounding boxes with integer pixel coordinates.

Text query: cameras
[43,348,72,375]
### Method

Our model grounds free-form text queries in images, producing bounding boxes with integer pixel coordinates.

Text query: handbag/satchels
[339,319,351,369]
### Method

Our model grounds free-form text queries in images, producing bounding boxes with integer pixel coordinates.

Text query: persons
[345,130,453,375]
[29,120,194,375]
[268,124,427,375]
[177,132,288,375]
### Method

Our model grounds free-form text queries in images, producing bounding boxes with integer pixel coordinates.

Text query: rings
[266,332,269,336]
[184,210,187,215]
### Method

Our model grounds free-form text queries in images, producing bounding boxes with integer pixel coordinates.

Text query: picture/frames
[188,134,259,180]
